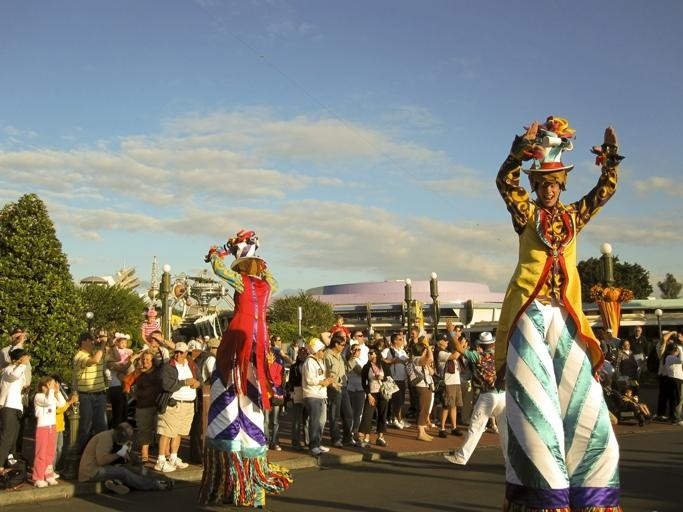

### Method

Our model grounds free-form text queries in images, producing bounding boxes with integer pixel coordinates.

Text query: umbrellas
[81,275,107,286]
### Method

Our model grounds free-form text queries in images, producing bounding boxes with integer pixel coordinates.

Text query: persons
[267,314,513,473]
[207,232,294,509]
[594,325,683,428]
[73,310,217,494]
[1,329,78,490]
[496,116,620,511]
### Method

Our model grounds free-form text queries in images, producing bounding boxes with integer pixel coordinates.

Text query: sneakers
[153,460,176,472]
[105,479,130,495]
[444,454,465,464]
[46,477,58,485]
[658,414,668,421]
[168,458,189,469]
[318,445,329,452]
[33,480,48,488]
[673,418,683,426]
[292,445,309,450]
[271,444,281,451]
[332,441,343,447]
[439,430,447,437]
[393,420,403,429]
[451,428,462,435]
[376,438,388,447]
[354,440,371,448]
[158,479,175,490]
[309,447,321,455]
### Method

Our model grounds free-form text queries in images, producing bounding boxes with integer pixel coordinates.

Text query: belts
[175,400,194,403]
[79,391,105,395]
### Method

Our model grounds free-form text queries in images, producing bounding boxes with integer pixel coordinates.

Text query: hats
[115,422,134,440]
[187,340,202,352]
[175,342,187,352]
[475,332,496,344]
[147,309,157,317]
[668,343,676,350]
[308,338,325,355]
[606,329,612,333]
[436,333,448,341]
[113,332,131,343]
[209,339,220,348]
[10,328,28,337]
[231,244,271,274]
[320,332,331,346]
[522,146,574,173]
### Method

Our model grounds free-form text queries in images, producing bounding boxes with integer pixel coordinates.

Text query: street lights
[654,309,666,339]
[159,264,174,341]
[592,244,634,341]
[402,278,415,342]
[429,272,441,355]
[295,304,305,339]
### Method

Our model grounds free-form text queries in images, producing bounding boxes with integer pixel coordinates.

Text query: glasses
[399,339,402,341]
[320,349,324,352]
[353,349,360,351]
[455,330,461,332]
[359,335,363,337]
[278,340,281,341]
[341,344,346,346]
[368,352,373,355]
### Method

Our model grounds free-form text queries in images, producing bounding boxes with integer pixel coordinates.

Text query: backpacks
[647,345,668,372]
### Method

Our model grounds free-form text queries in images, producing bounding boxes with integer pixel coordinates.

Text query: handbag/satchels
[293,387,304,403]
[381,386,392,400]
[272,387,284,406]
[432,375,444,405]
[167,398,177,406]
[0,455,26,488]
[327,388,336,402]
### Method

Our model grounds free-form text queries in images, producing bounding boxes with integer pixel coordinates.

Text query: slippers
[416,437,433,441]
[416,434,433,440]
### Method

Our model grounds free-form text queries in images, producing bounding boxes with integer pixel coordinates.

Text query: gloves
[116,440,128,457]
